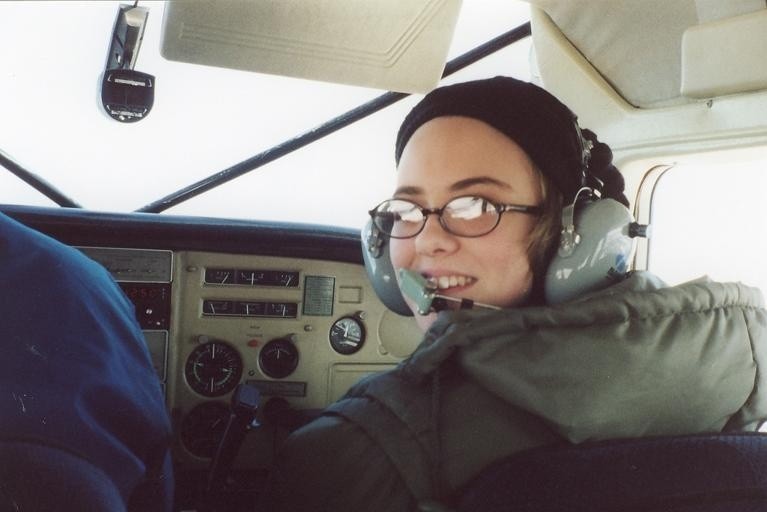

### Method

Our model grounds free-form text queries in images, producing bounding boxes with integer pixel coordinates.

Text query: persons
[1,210,176,511]
[269,74,767,512]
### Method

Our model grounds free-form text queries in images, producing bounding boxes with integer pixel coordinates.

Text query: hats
[395,77,630,209]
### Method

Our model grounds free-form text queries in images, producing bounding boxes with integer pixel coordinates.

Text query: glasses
[369,195,542,238]
